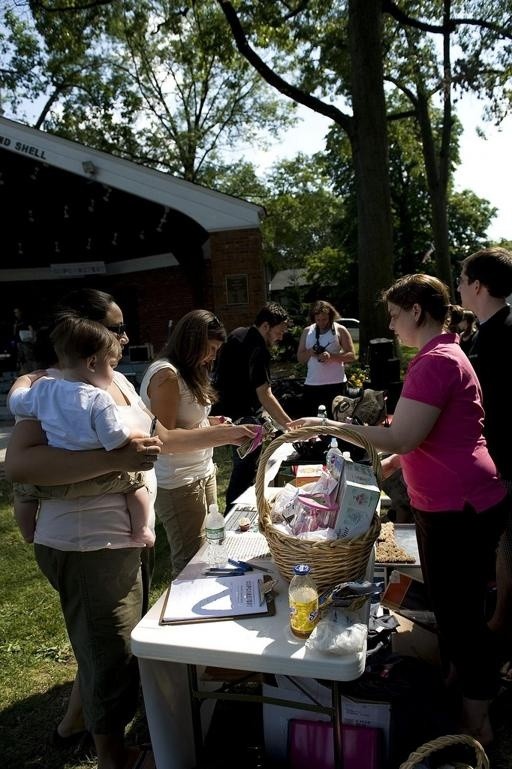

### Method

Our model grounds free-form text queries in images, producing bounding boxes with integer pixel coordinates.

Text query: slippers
[120,744,152,768]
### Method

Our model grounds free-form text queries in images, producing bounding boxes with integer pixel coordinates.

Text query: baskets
[255,425,382,595]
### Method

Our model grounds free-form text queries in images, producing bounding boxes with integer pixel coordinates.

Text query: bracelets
[321,417,328,426]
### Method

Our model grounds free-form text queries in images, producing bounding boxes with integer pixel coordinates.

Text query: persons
[5,310,160,548]
[2,289,265,768]
[284,271,510,769]
[208,300,295,518]
[455,245,511,487]
[296,298,356,414]
[137,306,238,575]
[457,310,477,357]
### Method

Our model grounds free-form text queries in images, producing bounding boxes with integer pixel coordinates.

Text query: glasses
[104,321,133,337]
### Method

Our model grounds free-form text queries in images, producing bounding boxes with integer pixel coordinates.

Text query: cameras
[312,343,325,354]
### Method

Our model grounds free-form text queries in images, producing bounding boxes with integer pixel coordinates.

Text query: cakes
[239,517,250,531]
[376,522,416,565]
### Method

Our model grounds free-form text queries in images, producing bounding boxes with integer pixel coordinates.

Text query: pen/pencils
[150,415,159,438]
[202,559,273,576]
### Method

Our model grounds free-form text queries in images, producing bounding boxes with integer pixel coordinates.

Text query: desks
[130,441,381,769]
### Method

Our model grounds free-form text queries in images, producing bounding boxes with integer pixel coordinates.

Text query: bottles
[204,504,228,573]
[326,438,343,468]
[288,565,319,638]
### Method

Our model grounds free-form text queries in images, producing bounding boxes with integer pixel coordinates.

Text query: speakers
[129,345,149,363]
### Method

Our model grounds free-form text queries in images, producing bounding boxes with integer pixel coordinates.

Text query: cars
[334,318,360,342]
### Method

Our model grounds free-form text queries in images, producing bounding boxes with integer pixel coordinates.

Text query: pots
[293,439,327,457]
